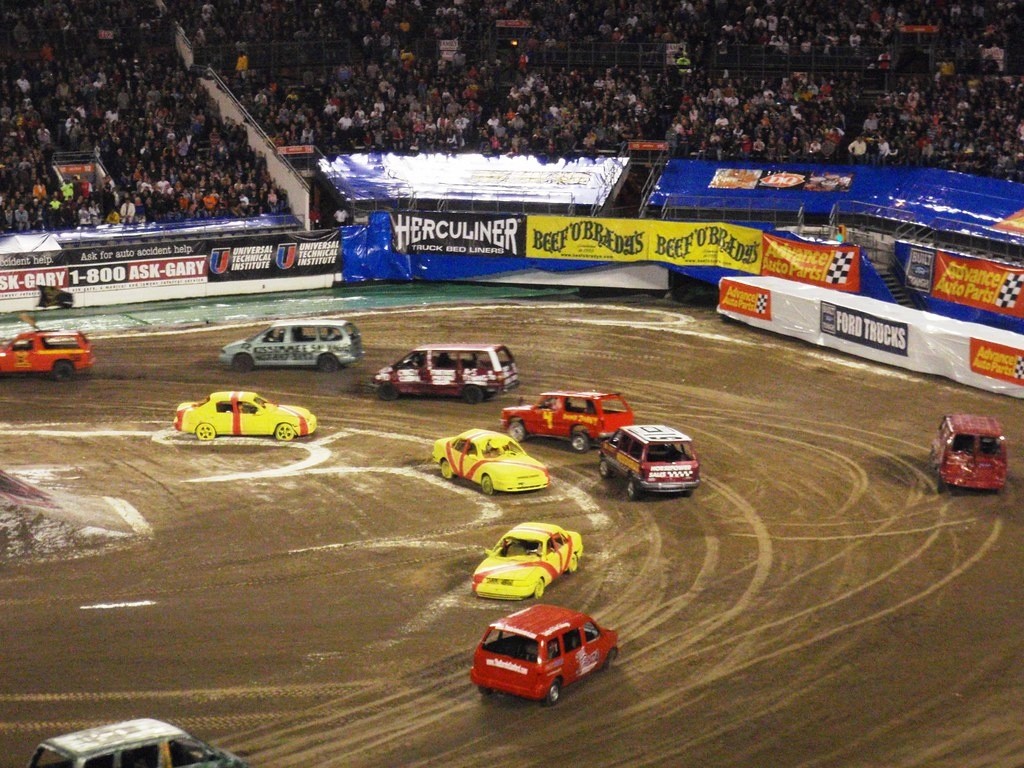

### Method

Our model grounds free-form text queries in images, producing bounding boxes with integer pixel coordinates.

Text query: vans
[598,425,700,502]
[930,414,1008,495]
[371,344,519,405]
[218,319,364,373]
[471,606,618,706]
[26,716,247,768]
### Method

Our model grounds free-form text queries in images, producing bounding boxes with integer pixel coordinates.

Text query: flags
[35,282,73,310]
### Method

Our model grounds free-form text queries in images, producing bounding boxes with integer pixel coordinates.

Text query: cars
[472,522,584,601]
[174,390,316,442]
[499,390,634,453]
[431,428,550,496]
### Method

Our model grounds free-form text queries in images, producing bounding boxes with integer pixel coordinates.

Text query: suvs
[0,330,96,383]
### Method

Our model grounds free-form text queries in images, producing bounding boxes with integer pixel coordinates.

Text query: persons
[299,0,641,160]
[1,0,300,230]
[332,203,349,227]
[237,402,253,414]
[628,1,1023,184]
[268,330,284,342]
[406,354,423,366]
